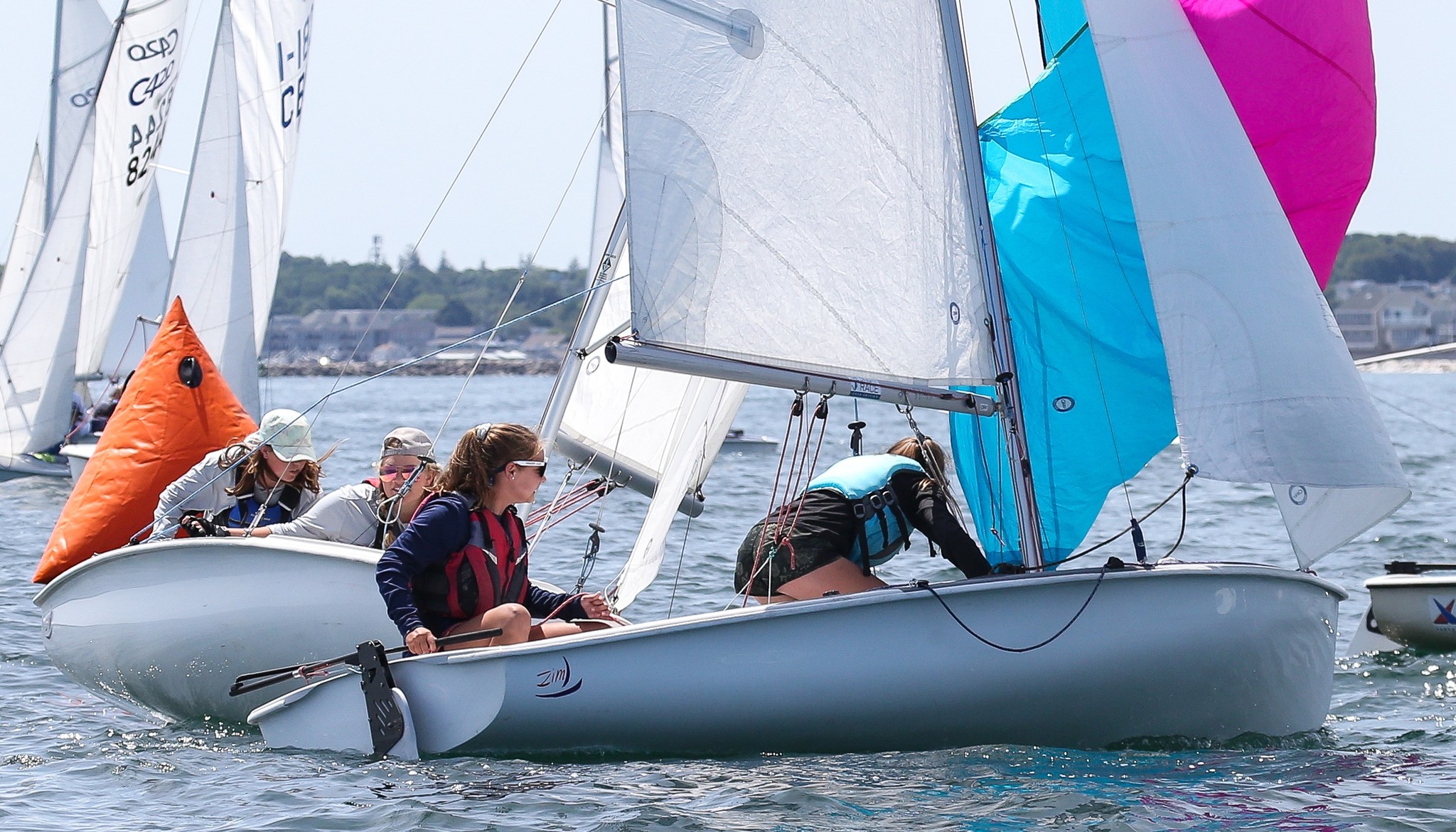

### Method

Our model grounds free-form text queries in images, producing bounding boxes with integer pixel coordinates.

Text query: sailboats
[222,0,1412,763]
[31,0,775,734]
[0,0,309,487]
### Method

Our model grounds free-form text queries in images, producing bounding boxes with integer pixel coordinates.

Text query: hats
[381,426,435,462]
[261,408,318,463]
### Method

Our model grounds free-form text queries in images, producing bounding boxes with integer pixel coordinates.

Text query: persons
[178,427,437,548]
[64,384,122,437]
[375,424,612,656]
[734,434,997,605]
[147,408,322,543]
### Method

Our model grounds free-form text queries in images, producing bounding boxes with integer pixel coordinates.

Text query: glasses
[378,464,422,484]
[493,456,549,477]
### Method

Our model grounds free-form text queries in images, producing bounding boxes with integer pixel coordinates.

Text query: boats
[1363,560,1456,653]
